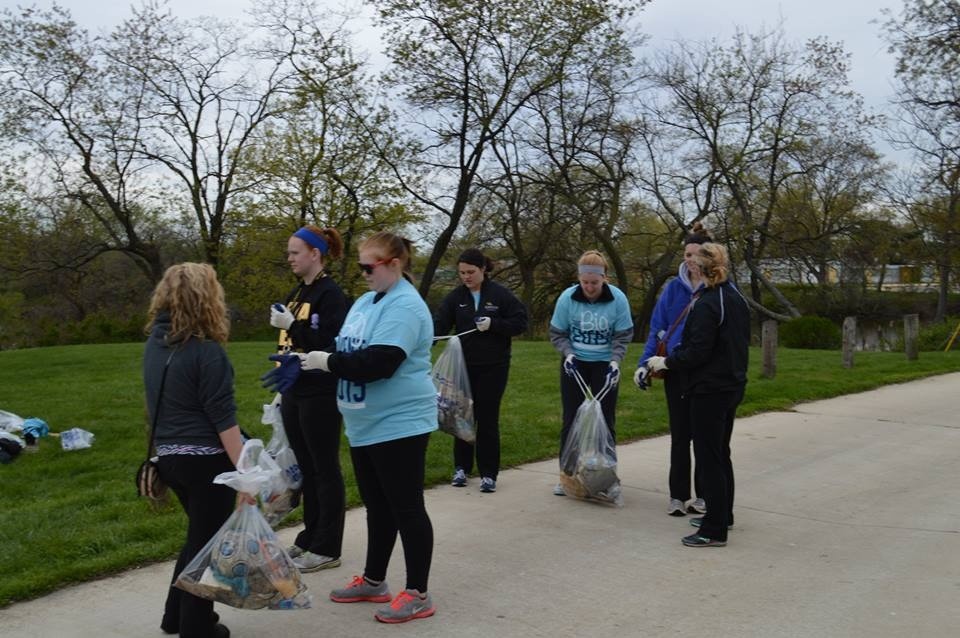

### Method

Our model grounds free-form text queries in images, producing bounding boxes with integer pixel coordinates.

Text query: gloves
[608,361,620,391]
[633,366,651,390]
[300,351,333,373]
[563,354,579,377]
[261,352,306,394]
[269,303,295,330]
[475,316,491,332]
[643,356,669,372]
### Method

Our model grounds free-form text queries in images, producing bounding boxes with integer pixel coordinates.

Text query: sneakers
[288,544,305,558]
[689,518,733,530]
[681,533,727,547]
[666,498,686,515]
[292,551,341,573]
[479,477,496,492]
[330,576,392,603]
[688,497,707,514]
[451,469,468,487]
[374,589,436,623]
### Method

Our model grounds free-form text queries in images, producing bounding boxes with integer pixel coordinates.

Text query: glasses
[357,257,396,274]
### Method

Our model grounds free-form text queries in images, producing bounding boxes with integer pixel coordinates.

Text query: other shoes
[216,624,231,638]
[553,482,567,495]
[165,612,219,634]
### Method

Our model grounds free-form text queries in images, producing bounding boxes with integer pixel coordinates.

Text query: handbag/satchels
[136,456,168,500]
[649,341,666,379]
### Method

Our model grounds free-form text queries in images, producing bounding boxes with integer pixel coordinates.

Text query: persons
[143,262,256,638]
[633,221,713,516]
[433,248,527,492]
[270,225,349,574]
[643,241,752,547]
[300,231,438,623]
[548,250,633,495]
